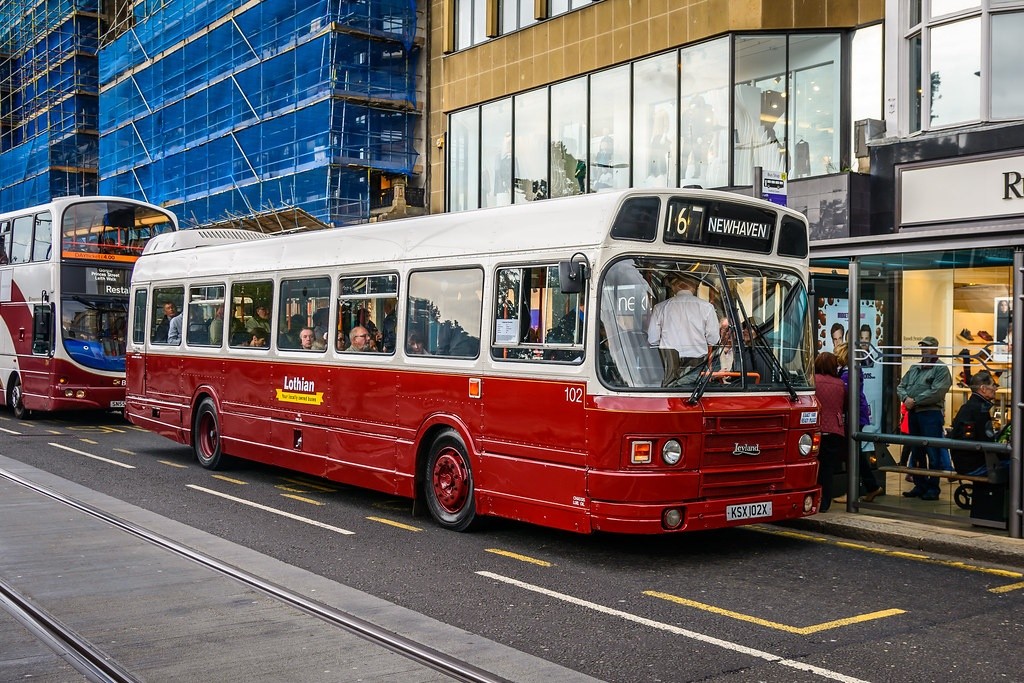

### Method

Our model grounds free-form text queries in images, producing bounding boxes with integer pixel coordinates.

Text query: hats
[918,337,938,347]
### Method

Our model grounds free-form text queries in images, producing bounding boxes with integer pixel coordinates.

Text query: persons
[949,369,1011,477]
[996,300,1012,352]
[832,343,884,504]
[832,323,874,367]
[86,238,141,255]
[154,296,479,357]
[647,273,721,372]
[558,295,608,339]
[814,352,846,512]
[709,317,772,385]
[896,337,957,501]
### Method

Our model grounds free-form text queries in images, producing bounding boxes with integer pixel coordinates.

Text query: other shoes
[903,485,923,496]
[820,508,826,512]
[905,476,913,482]
[834,497,847,503]
[922,488,941,499]
[862,487,883,503]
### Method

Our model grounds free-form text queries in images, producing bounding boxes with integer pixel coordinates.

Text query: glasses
[354,334,370,338]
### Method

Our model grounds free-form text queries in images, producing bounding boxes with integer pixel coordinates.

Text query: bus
[124,183,826,533]
[0,194,180,421]
